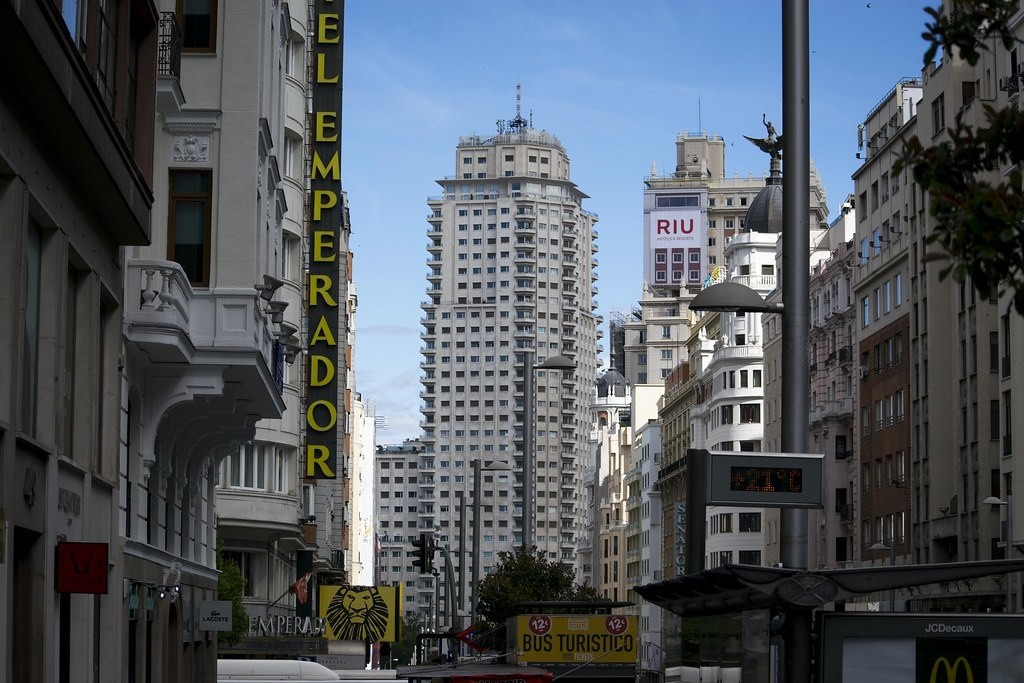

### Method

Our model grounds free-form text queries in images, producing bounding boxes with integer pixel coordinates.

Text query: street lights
[523,350,576,557]
[689,282,813,683]
[471,458,512,660]
[870,541,895,613]
[444,546,472,627]
[983,496,1013,615]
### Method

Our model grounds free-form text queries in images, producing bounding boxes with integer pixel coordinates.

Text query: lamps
[272,323,298,345]
[858,252,870,260]
[253,274,285,301]
[878,236,891,244]
[282,345,303,364]
[890,227,903,235]
[265,301,289,323]
[870,242,881,248]
[846,261,860,267]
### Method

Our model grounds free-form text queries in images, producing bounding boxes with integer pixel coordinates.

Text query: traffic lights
[411,534,428,574]
